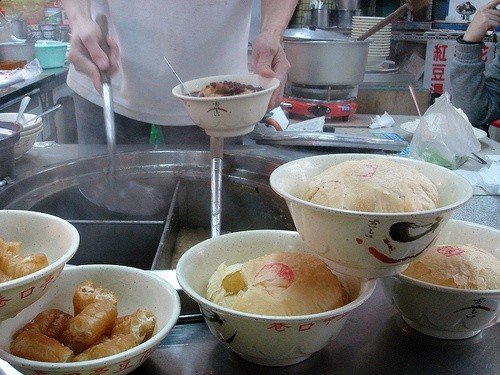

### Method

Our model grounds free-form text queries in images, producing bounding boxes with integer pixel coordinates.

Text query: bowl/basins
[176,229,375,366]
[0,208,81,323]
[269,153,474,278]
[383,218,500,340]
[172,73,280,138]
[0,113,44,180]
[35,45,67,70]
[0,263,181,375]
[350,16,392,71]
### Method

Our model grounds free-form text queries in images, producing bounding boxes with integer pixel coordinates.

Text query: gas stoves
[279,83,359,123]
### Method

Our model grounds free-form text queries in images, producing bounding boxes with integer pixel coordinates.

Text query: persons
[450,0,500,128]
[62,1,300,145]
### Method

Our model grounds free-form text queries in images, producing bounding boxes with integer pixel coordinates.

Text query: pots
[310,8,364,28]
[0,43,36,68]
[1,20,27,42]
[281,25,374,87]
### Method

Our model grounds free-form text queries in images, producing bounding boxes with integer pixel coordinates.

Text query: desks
[0,68,58,145]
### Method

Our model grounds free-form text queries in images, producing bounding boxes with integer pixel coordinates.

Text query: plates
[400,120,487,140]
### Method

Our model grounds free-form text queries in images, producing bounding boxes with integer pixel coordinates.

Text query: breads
[398,244,500,290]
[297,158,440,213]
[207,250,351,316]
